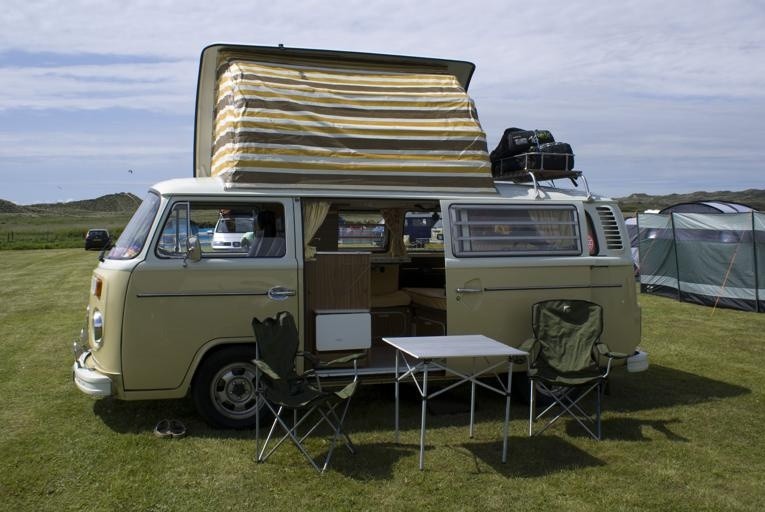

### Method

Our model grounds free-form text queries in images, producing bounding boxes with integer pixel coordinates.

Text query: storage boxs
[371,290,412,346]
[372,254,411,292]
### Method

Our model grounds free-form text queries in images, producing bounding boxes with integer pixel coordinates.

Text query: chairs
[509,299,613,439]
[250,210,270,256]
[270,217,286,256]
[252,312,366,475]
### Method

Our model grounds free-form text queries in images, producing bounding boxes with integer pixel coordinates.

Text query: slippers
[153,418,187,440]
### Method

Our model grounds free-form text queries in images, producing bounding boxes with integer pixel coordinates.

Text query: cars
[85,229,112,250]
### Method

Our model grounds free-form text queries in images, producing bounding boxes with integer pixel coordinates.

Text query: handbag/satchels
[489,127,575,176]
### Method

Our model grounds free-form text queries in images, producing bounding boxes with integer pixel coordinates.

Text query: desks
[380,335,530,470]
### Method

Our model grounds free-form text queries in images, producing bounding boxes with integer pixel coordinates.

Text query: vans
[207,217,254,252]
[73,44,649,429]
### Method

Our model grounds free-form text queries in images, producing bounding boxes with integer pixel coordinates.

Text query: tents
[624,197,765,316]
